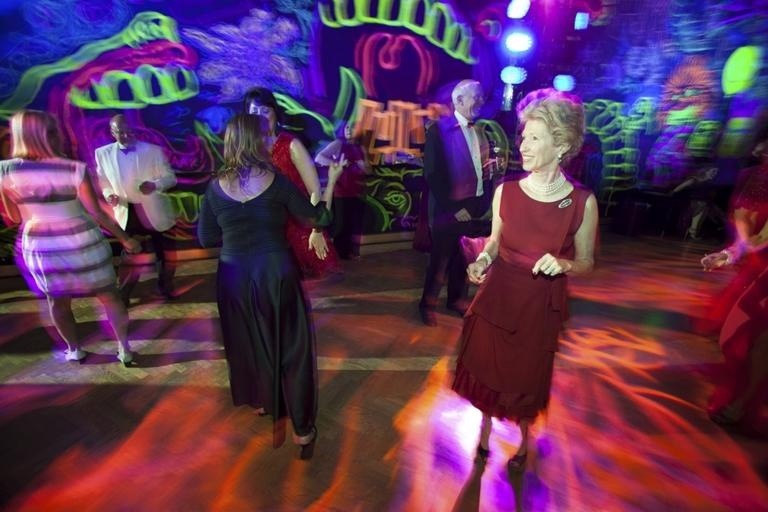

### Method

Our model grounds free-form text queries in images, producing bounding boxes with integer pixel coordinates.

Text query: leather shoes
[419,300,474,327]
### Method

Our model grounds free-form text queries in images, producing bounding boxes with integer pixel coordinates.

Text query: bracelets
[476,252,492,267]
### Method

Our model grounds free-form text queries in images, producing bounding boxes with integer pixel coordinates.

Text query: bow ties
[468,122,474,128]
[120,146,136,156]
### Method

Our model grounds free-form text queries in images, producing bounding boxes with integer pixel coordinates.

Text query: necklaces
[527,173,566,195]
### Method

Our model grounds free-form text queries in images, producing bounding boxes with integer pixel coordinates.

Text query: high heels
[292,425,317,459]
[479,442,490,463]
[116,351,133,367]
[253,407,267,415]
[508,451,527,473]
[64,349,87,361]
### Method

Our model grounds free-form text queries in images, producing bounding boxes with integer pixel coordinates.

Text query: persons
[315,120,370,259]
[95,115,178,306]
[451,89,598,470]
[669,142,768,436]
[1,110,136,366]
[419,79,498,327]
